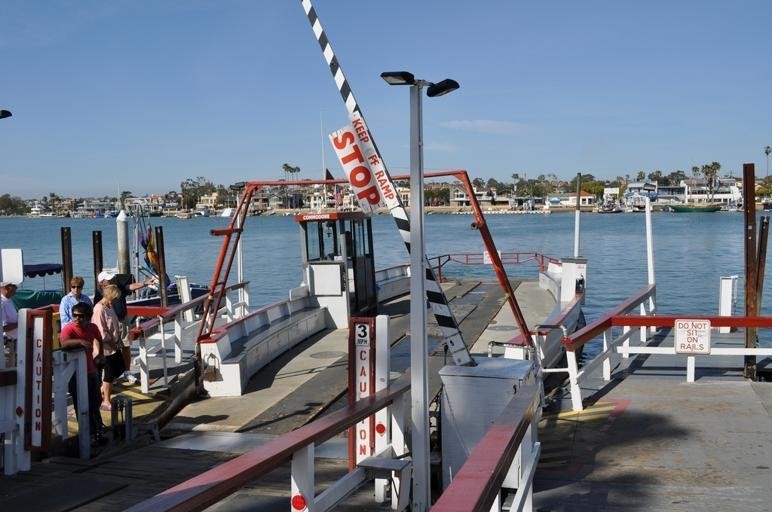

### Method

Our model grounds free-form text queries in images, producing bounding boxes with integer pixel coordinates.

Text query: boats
[597,209,623,213]
[632,209,654,213]
[0,170,587,512]
[670,205,722,212]
[736,206,744,212]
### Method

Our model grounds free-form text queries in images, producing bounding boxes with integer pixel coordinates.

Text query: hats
[96,270,116,284]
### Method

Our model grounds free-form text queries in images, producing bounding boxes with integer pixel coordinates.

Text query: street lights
[380,71,461,512]
[765,146,772,180]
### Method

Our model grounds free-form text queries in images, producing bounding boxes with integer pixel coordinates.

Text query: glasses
[70,285,82,289]
[71,313,87,318]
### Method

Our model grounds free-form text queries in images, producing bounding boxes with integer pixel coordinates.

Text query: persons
[1,278,25,345]
[91,283,125,413]
[60,301,108,442]
[58,275,95,326]
[96,271,158,386]
[143,275,159,299]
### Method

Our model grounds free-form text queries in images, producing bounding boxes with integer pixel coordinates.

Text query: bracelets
[143,280,148,288]
